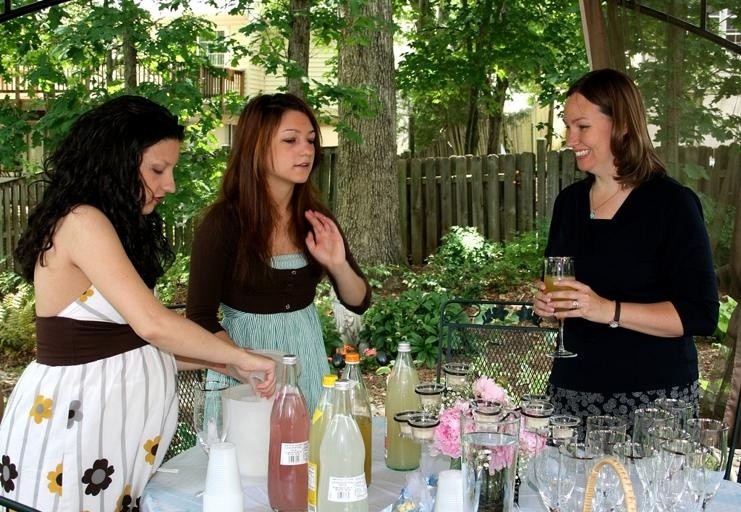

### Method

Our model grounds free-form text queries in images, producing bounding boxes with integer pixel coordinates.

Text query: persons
[0,94,277,512]
[187,93,373,440]
[531,69,719,456]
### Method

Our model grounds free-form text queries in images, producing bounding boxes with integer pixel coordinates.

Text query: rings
[572,300,579,309]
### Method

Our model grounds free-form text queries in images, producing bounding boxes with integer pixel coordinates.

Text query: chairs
[438,298,567,404]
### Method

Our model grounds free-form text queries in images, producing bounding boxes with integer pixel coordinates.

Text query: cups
[435,469,463,512]
[461,405,521,512]
[394,362,581,445]
[202,440,244,512]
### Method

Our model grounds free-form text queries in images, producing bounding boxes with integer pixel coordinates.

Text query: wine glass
[534,398,709,511]
[193,380,231,500]
[543,255,580,360]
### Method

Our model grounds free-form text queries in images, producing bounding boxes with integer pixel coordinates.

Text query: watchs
[609,301,621,328]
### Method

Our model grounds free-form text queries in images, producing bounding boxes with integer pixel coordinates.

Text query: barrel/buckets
[223,382,283,478]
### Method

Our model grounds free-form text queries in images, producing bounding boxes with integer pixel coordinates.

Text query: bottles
[311,352,373,512]
[268,354,310,512]
[386,340,422,470]
